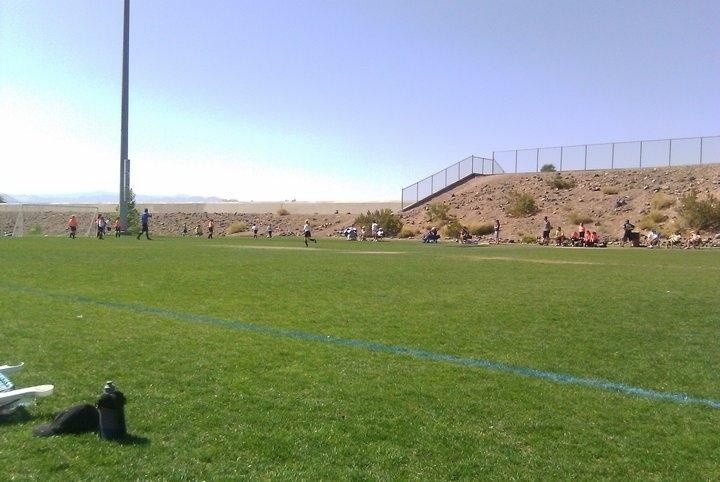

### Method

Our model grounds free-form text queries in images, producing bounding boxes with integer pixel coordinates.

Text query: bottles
[95,379,127,439]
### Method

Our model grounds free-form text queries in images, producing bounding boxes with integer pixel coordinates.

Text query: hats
[34,404,100,437]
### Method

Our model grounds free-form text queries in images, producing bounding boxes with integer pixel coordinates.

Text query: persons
[460,228,471,244]
[267,224,273,238]
[423,226,437,244]
[137,209,152,239]
[345,224,357,240]
[184,219,214,238]
[645,228,701,249]
[96,215,121,239]
[620,219,636,247]
[493,219,500,244]
[543,216,598,247]
[68,215,78,238]
[302,220,316,247]
[359,220,384,242]
[251,224,258,238]
